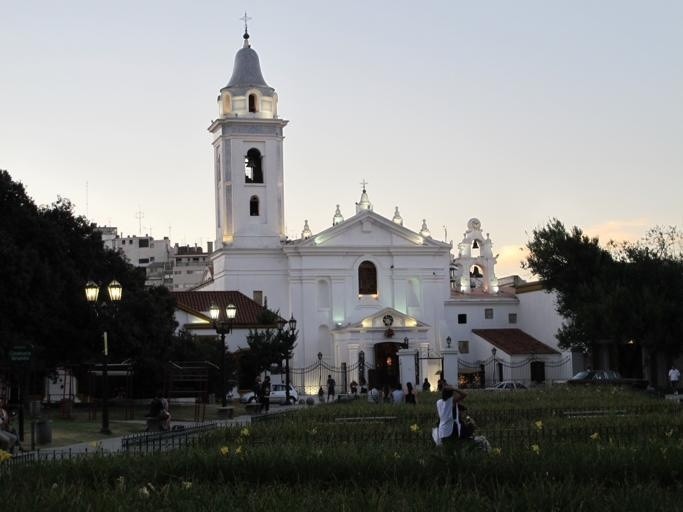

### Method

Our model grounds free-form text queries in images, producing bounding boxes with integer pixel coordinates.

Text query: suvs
[238,384,299,405]
[567,369,615,388]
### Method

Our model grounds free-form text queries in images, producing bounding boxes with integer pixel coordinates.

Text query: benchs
[245,403,262,414]
[216,407,234,420]
[337,393,354,401]
[146,416,161,432]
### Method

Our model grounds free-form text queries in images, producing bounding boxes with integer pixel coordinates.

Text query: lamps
[446,336,451,348]
[403,337,409,349]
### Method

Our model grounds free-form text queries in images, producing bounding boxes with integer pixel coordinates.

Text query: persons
[259,376,271,413]
[255,375,262,403]
[147,393,166,430]
[668,364,680,395]
[350,377,446,405]
[161,392,172,426]
[431,385,468,448]
[0,397,16,452]
[326,375,335,402]
[319,385,324,400]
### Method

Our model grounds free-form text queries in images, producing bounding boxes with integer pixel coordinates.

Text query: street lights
[275,312,297,405]
[317,351,323,387]
[208,300,237,407]
[84,278,125,435]
[490,347,498,384]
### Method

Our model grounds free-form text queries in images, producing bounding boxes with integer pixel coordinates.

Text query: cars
[485,380,527,392]
